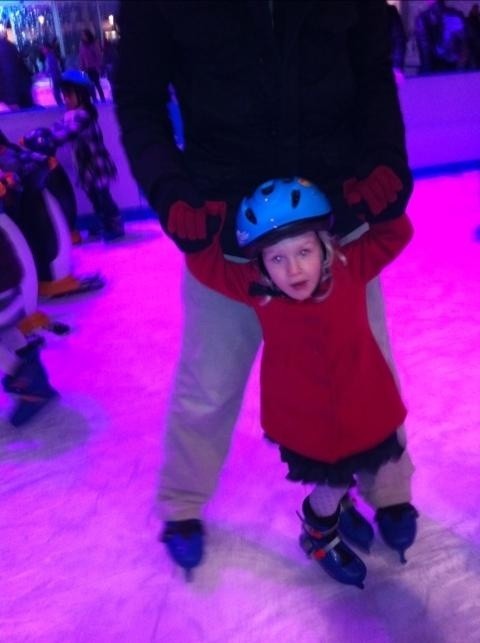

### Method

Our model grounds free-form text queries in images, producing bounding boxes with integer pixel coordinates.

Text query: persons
[0,34,38,110]
[42,69,125,245]
[385,1,406,88]
[23,28,113,106]
[466,4,479,70]
[415,0,468,75]
[112,0,410,570]
[165,164,417,588]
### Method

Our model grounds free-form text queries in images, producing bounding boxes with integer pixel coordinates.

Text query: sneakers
[377,504,417,552]
[336,493,375,548]
[161,519,203,569]
[298,498,367,585]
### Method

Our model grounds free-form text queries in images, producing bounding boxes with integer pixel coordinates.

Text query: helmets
[237,180,336,255]
